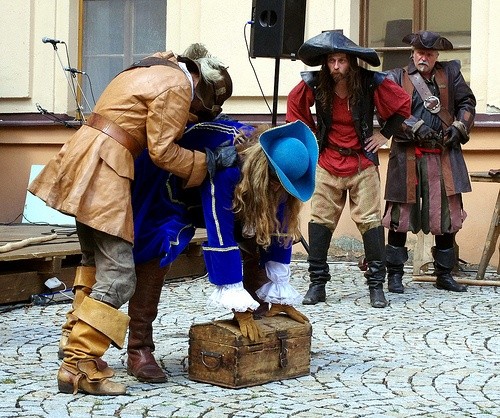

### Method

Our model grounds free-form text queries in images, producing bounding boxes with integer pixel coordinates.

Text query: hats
[176,42,232,113]
[402,31,455,50]
[257,119,319,203]
[296,31,381,67]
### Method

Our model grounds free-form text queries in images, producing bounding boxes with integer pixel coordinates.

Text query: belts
[326,144,363,176]
[420,140,444,149]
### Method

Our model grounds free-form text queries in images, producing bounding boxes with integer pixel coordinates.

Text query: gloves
[415,124,438,141]
[265,303,310,324]
[443,126,463,150]
[233,311,263,342]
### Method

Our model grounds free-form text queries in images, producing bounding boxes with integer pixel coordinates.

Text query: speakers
[250,0,307,61]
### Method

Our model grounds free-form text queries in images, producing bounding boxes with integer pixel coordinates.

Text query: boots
[58,266,98,359]
[302,222,332,305]
[361,225,388,308]
[385,244,409,293]
[236,243,272,308]
[55,295,131,396]
[431,246,467,292]
[126,261,168,383]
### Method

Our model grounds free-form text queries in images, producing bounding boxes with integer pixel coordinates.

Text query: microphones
[42,37,65,44]
[63,66,87,75]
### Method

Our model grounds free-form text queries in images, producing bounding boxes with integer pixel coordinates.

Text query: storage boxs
[189,314,312,388]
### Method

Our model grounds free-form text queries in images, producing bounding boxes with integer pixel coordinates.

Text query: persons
[127,118,319,382]
[287,30,412,308]
[380,31,476,295]
[27,44,238,397]
